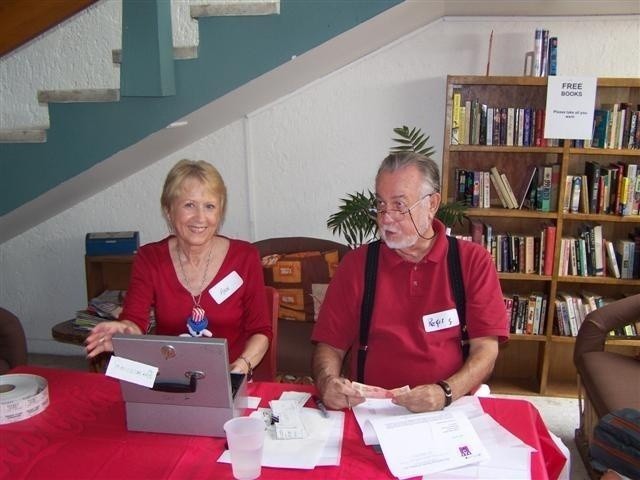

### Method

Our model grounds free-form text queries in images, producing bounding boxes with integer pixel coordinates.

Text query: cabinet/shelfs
[440,75,640,398]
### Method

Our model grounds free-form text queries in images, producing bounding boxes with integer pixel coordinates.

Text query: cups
[223,416,267,480]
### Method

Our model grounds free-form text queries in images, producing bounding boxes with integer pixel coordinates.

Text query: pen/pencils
[311,394,330,418]
[341,373,350,411]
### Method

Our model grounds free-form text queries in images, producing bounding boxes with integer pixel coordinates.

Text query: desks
[0,365,549,480]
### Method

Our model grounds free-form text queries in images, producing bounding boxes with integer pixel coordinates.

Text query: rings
[99,338,104,342]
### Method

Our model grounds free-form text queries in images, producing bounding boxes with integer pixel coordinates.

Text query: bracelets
[437,380,452,406]
[240,357,253,376]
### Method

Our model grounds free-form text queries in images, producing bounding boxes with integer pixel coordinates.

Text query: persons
[310,152,509,414]
[86,168,273,374]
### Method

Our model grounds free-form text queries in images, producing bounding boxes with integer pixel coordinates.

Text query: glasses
[367,194,434,221]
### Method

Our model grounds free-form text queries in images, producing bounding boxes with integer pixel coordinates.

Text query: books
[74,289,156,331]
[531,27,557,78]
[558,223,640,280]
[563,160,640,216]
[572,103,640,149]
[555,290,640,337]
[455,165,561,213]
[451,93,558,147]
[453,219,557,276]
[502,291,548,335]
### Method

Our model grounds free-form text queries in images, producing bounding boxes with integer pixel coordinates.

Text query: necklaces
[176,238,213,337]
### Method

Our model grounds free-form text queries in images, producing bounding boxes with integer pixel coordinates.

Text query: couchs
[253,237,351,378]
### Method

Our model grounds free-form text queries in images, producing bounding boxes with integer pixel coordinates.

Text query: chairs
[0,307,28,374]
[573,295,640,480]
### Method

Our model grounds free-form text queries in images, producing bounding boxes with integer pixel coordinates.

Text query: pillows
[261,249,339,323]
